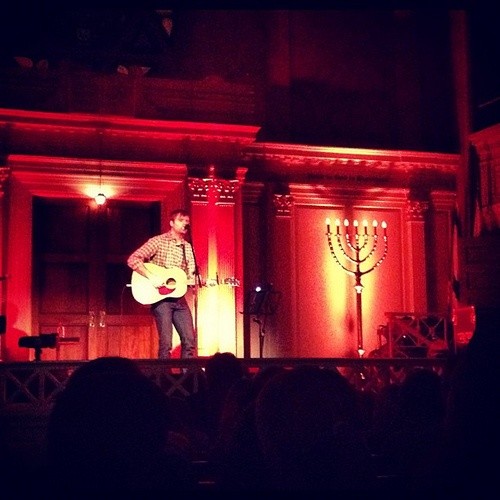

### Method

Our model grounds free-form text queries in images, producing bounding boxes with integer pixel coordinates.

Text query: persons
[12,356,194,500]
[185,352,499,499]
[126,210,196,359]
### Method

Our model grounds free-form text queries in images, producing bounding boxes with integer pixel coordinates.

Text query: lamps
[95,160,106,205]
[325,218,388,391]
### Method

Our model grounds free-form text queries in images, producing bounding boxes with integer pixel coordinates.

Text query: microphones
[184,224,192,239]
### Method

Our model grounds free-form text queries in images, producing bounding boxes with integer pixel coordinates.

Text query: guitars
[131,262,241,305]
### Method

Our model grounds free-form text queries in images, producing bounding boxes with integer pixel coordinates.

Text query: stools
[20,335,55,362]
